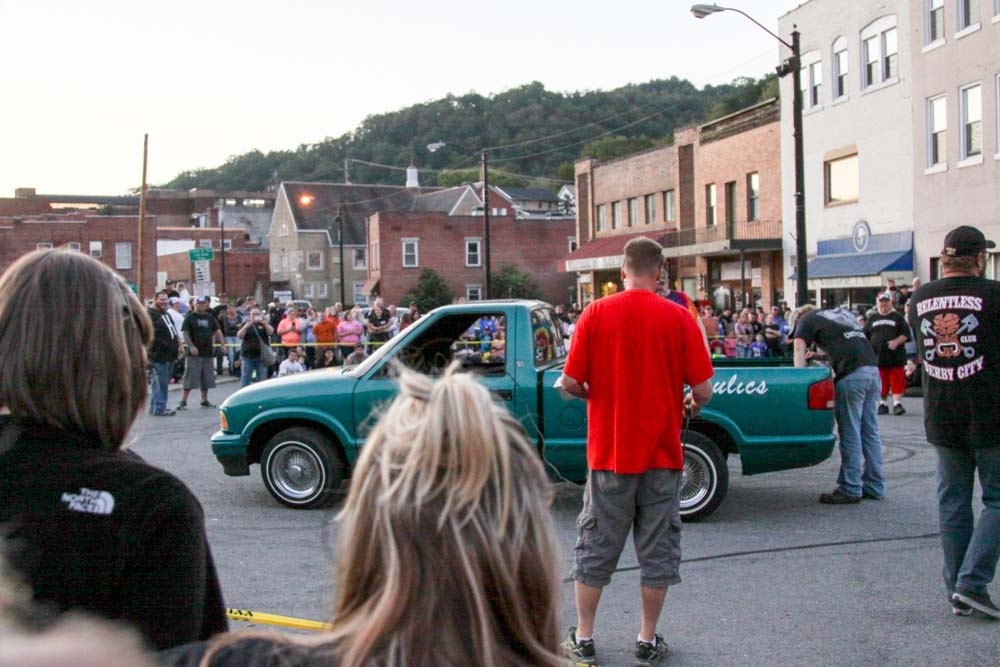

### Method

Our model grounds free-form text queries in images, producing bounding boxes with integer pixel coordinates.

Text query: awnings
[557,227,676,273]
[787,230,916,292]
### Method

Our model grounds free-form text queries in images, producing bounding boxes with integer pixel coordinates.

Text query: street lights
[689,3,811,306]
[426,142,491,299]
[301,195,346,311]
[190,213,226,298]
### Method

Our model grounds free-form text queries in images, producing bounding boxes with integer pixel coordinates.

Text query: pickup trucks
[208,300,836,524]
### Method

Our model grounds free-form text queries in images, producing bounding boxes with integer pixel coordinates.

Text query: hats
[877,292,890,301]
[193,296,207,304]
[941,226,996,257]
[788,304,816,338]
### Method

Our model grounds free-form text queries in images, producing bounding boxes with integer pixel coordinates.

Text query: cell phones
[254,316,263,320]
[292,322,295,326]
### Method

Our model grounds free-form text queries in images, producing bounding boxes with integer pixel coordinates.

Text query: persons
[656,268,921,416]
[201,372,567,667]
[908,224,1000,620]
[560,235,714,667]
[146,278,581,415]
[788,304,886,505]
[0,247,230,667]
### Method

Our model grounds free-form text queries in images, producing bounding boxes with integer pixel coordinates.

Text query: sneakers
[633,633,673,667]
[560,626,598,663]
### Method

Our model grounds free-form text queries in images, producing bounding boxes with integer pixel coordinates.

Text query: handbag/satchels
[260,342,276,365]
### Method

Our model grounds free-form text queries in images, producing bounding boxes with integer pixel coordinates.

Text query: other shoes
[200,401,216,408]
[176,400,187,410]
[893,403,905,415]
[952,587,1000,619]
[820,489,861,504]
[149,409,176,416]
[878,403,890,414]
